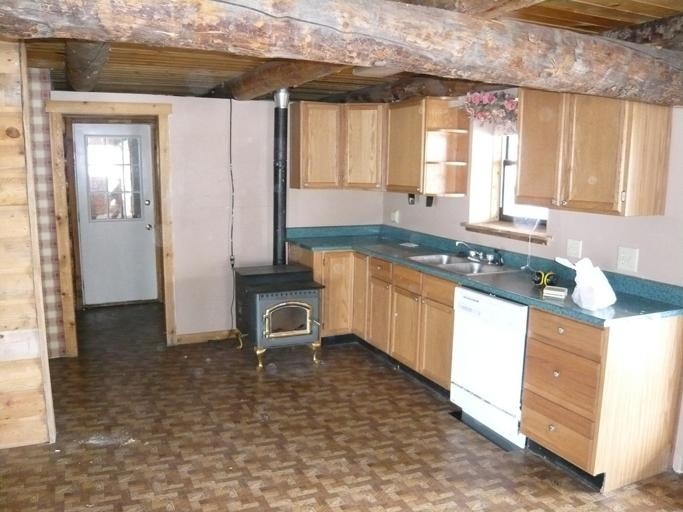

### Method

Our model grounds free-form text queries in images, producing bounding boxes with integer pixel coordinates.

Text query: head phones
[531,270,558,287]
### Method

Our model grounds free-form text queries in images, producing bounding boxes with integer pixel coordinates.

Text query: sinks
[406,253,468,265]
[436,263,523,276]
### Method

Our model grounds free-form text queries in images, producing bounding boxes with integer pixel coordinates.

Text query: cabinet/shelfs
[299,97,470,196]
[515,88,630,216]
[311,250,457,391]
[518,305,609,495]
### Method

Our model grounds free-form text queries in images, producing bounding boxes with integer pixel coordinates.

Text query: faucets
[456,241,483,258]
